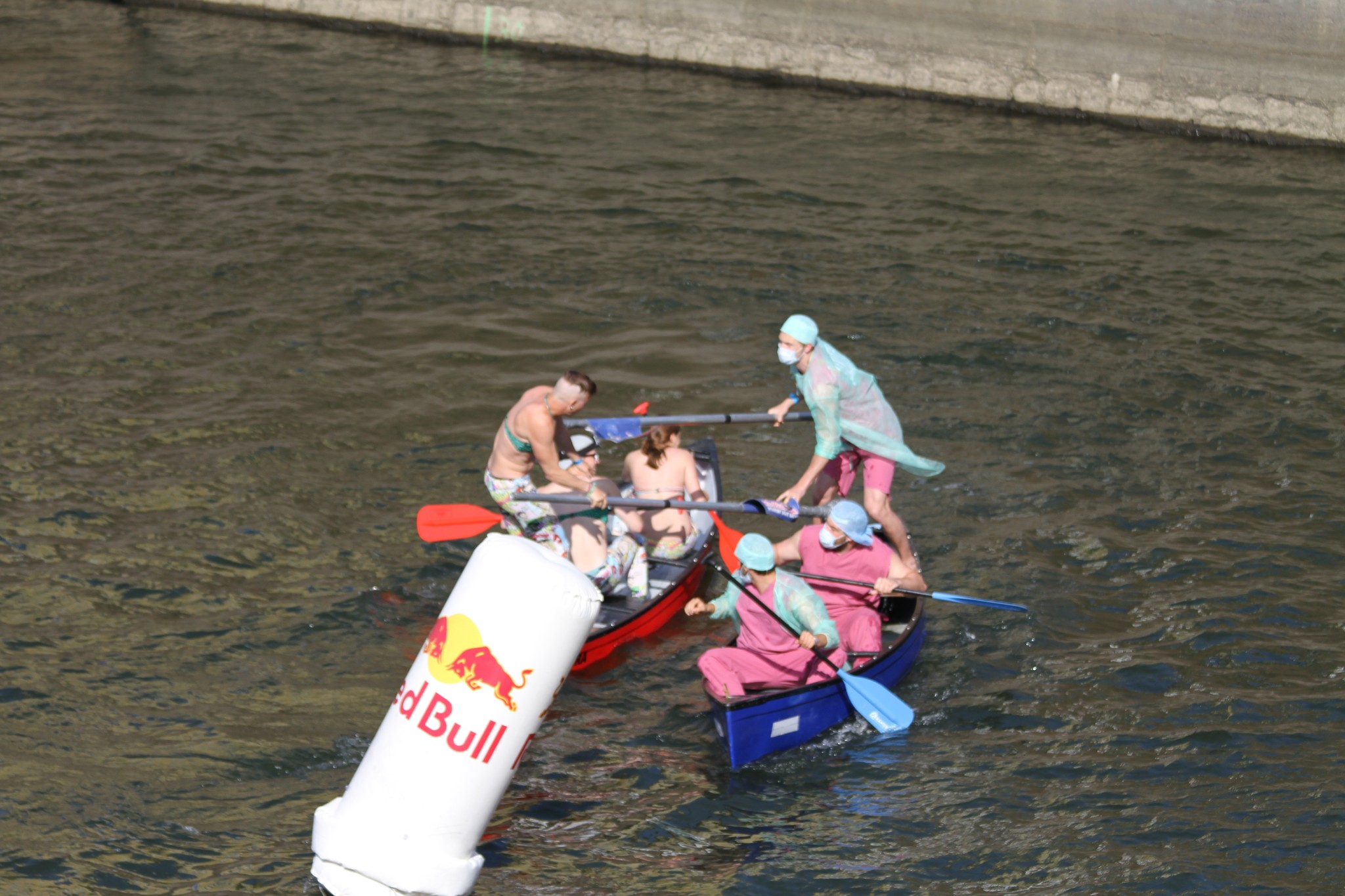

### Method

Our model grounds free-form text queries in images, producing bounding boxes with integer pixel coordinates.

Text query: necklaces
[545,393,555,418]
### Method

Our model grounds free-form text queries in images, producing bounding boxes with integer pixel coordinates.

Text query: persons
[771,500,928,671]
[684,533,848,706]
[483,370,650,604]
[767,313,945,572]
[622,425,709,560]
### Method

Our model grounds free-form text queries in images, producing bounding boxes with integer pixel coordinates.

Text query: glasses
[581,452,599,463]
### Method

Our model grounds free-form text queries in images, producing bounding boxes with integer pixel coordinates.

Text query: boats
[569,439,724,674]
[699,516,928,772]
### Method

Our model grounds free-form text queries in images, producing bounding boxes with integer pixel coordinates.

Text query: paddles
[695,549,915,732]
[414,500,512,543]
[783,566,1030,616]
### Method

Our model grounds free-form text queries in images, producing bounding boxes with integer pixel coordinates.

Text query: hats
[560,434,596,458]
[829,501,882,545]
[780,314,819,348]
[734,533,774,571]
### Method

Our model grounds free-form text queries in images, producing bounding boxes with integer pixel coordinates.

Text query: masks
[777,344,806,365]
[818,523,848,549]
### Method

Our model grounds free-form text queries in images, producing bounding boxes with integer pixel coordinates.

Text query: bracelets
[584,482,592,494]
[573,458,582,465]
[586,482,596,497]
[789,393,799,405]
[813,637,818,645]
